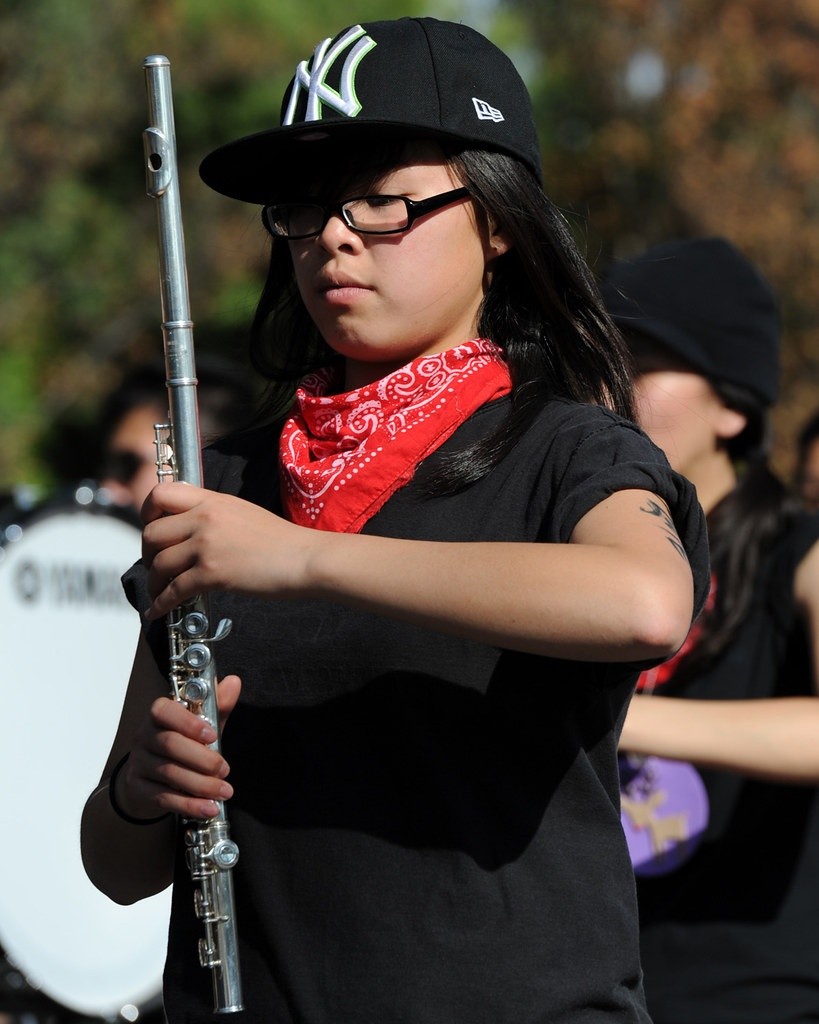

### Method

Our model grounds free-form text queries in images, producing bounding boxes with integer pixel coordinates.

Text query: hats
[198,15,545,205]
[596,235,787,406]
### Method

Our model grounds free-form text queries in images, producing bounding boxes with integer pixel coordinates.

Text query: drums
[0,489,174,1022]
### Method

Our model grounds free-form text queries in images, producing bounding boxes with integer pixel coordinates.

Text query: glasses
[261,185,468,239]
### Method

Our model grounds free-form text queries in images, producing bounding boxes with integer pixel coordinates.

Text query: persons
[80,14,710,1024]
[593,235,819,1022]
[0,319,252,1024]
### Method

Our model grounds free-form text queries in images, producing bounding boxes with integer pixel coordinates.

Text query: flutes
[140,53,243,1017]
